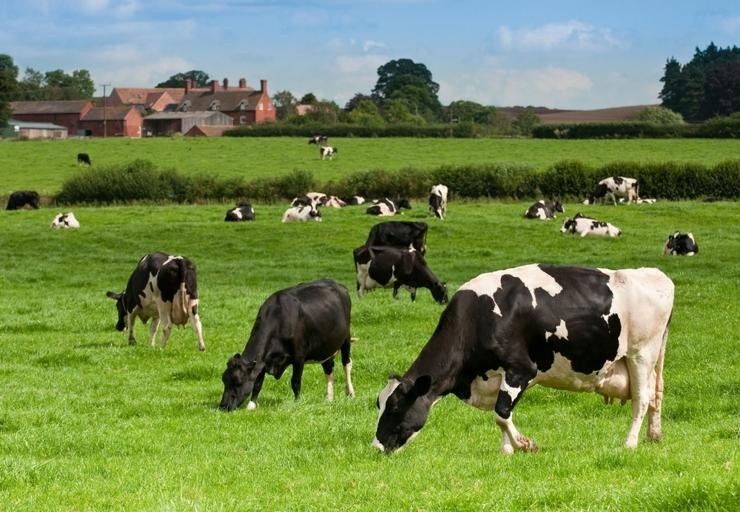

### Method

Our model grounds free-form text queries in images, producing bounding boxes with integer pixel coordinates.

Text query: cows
[103,251,210,354]
[225,200,256,220]
[6,190,41,210]
[219,278,357,413]
[75,150,95,167]
[280,184,449,224]
[525,175,656,242]
[662,231,698,256]
[371,263,677,458]
[352,220,450,305]
[50,210,86,232]
[308,133,338,162]
